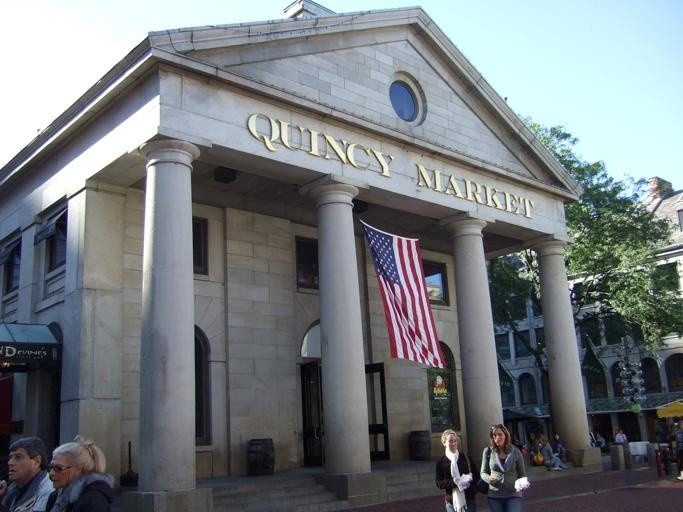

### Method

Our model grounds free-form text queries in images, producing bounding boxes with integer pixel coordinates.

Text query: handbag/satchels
[534,447,545,466]
[477,446,492,495]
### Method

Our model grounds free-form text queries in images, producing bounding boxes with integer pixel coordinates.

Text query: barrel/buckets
[249,438,275,475]
[615,440,631,468]
[410,429,431,461]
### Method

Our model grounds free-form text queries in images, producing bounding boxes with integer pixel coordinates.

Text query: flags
[358,221,448,371]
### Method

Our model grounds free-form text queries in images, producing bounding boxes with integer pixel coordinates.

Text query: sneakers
[676,470,683,482]
[551,467,568,473]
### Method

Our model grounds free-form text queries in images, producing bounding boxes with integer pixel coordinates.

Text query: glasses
[45,463,73,472]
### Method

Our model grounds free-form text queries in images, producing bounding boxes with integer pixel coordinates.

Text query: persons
[614,429,627,443]
[433,429,480,511]
[528,431,568,472]
[674,420,683,481]
[595,432,609,448]
[45,435,115,511]
[0,436,58,511]
[479,423,530,511]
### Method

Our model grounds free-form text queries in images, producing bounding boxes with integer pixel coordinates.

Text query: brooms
[119,440,139,486]
